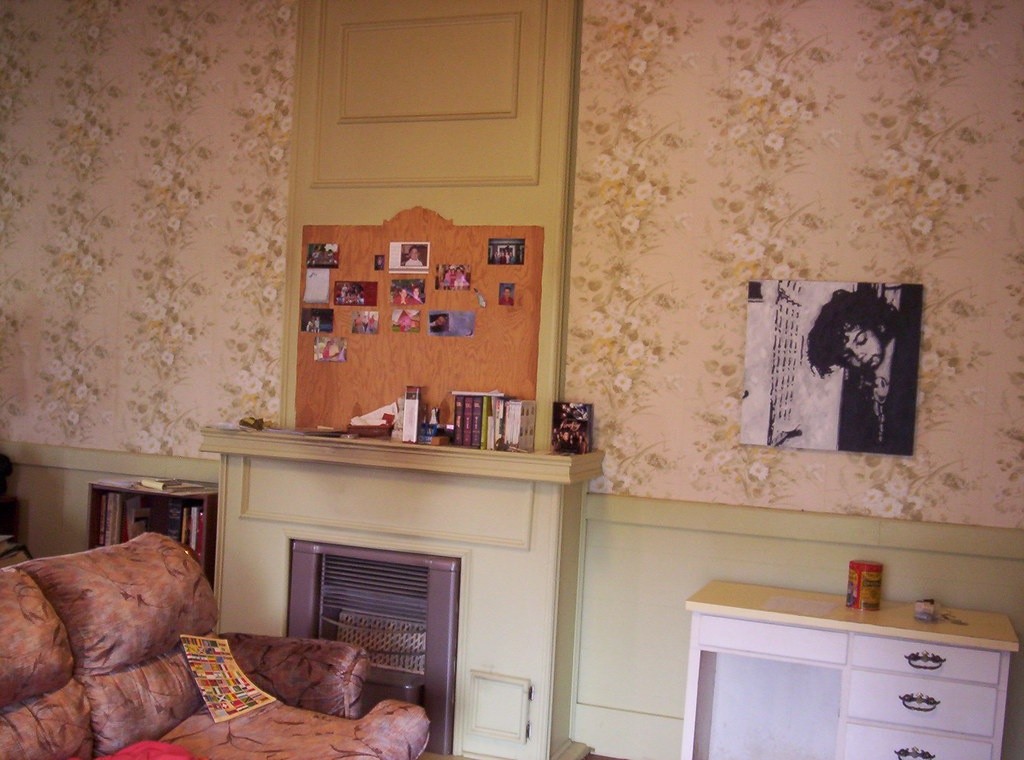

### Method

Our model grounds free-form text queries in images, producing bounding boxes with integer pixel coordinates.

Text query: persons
[430,316,446,332]
[499,287,513,305]
[399,322,410,332]
[307,247,321,267]
[341,283,360,305]
[804,289,899,425]
[442,268,463,290]
[406,248,424,266]
[327,248,337,265]
[354,314,376,334]
[307,315,319,329]
[322,340,331,358]
[491,249,517,265]
[393,285,423,305]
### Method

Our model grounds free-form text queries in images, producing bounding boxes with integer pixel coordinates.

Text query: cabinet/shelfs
[85,477,219,593]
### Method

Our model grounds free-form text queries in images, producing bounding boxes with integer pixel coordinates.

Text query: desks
[680,580,1020,760]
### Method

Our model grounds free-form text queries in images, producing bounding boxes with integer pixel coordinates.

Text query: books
[98,493,204,561]
[551,401,594,454]
[452,397,535,450]
[402,385,419,443]
[142,477,204,490]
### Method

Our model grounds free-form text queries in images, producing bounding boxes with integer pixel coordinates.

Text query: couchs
[0,533,431,760]
